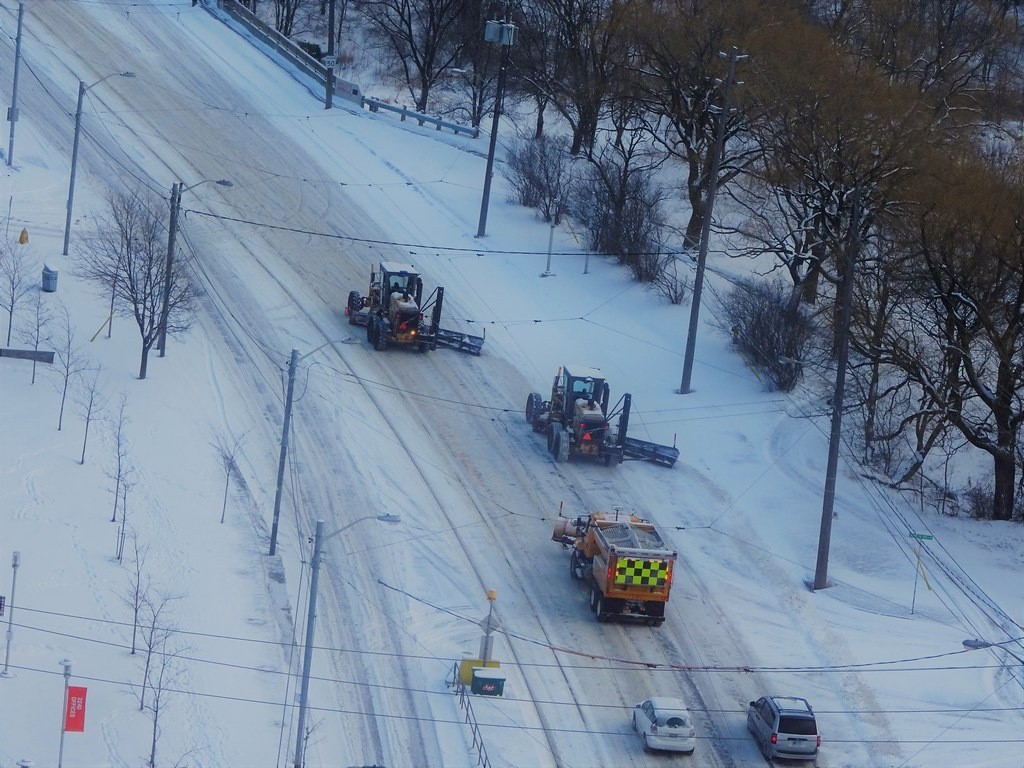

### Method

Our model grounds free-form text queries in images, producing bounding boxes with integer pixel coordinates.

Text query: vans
[746,695,821,762]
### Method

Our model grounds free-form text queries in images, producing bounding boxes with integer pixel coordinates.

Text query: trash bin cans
[42,264,58,292]
[470,667,505,695]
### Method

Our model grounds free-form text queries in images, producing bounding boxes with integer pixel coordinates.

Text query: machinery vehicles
[551,499,677,627]
[524,365,632,465]
[343,261,437,355]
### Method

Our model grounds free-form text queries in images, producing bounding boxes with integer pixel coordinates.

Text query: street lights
[156,178,235,358]
[267,337,363,557]
[674,44,754,395]
[293,512,400,768]
[61,69,138,258]
[473,0,517,237]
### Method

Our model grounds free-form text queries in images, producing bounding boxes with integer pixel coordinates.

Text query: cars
[631,696,697,756]
